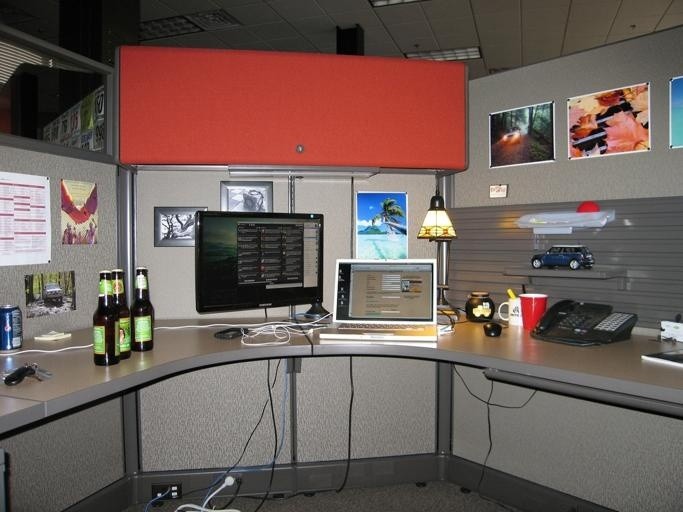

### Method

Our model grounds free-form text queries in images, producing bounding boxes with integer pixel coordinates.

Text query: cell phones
[215,327,248,339]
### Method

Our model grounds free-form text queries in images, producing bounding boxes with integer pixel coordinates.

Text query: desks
[1,314,681,443]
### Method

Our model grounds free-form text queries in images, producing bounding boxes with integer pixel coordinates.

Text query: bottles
[110,268,132,360]
[129,265,154,352]
[464,291,495,322]
[93,269,120,365]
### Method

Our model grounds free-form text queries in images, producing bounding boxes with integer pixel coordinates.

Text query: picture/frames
[219,181,273,213]
[153,206,208,247]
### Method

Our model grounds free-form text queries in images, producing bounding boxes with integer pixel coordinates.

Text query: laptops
[319,259,438,349]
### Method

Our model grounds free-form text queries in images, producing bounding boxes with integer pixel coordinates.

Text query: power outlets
[150,483,181,501]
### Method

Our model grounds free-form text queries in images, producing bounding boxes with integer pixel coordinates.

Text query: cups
[518,293,548,330]
[497,298,524,327]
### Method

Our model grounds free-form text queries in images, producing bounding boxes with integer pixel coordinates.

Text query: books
[34,330,72,340]
[641,349,682,368]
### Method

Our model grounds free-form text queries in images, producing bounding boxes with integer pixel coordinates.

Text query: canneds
[0,304,23,353]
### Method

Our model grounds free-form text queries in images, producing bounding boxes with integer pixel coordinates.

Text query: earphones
[308,328,314,334]
[272,325,276,328]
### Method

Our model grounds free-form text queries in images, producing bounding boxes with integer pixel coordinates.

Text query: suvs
[43,283,63,304]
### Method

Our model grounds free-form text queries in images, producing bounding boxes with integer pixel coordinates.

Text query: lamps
[417,173,457,241]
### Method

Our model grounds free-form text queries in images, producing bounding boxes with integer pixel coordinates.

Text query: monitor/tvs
[195,210,324,312]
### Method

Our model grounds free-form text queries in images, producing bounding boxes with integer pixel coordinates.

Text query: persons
[63,217,96,244]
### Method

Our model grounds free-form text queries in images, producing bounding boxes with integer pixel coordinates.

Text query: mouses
[483,323,503,337]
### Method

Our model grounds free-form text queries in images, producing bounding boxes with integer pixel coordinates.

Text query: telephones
[530,300,637,346]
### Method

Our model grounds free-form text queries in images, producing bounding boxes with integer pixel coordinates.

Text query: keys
[4,362,51,386]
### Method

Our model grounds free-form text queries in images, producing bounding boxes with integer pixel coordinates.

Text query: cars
[502,127,522,144]
